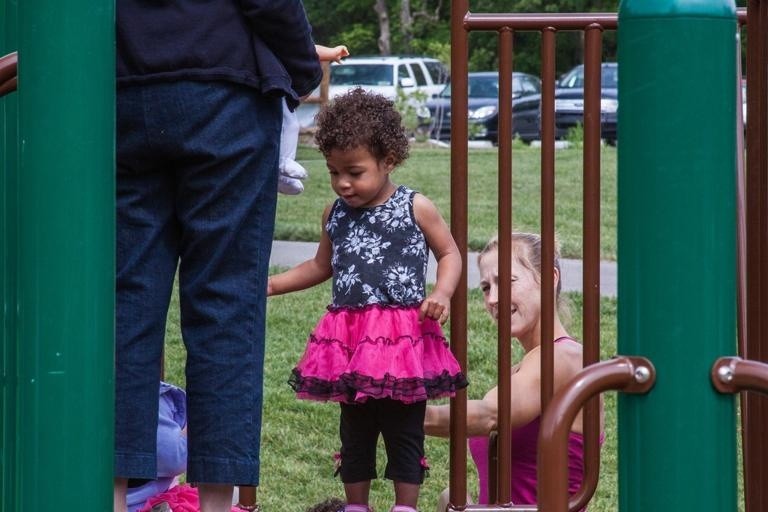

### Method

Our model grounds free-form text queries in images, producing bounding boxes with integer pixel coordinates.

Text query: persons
[267,86,463,512]
[424,232,605,512]
[115,0,307,512]
[278,40,350,196]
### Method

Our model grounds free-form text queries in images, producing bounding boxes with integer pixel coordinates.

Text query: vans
[328,56,446,115]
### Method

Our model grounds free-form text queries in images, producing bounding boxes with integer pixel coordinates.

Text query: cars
[416,72,541,142]
[556,61,618,136]
